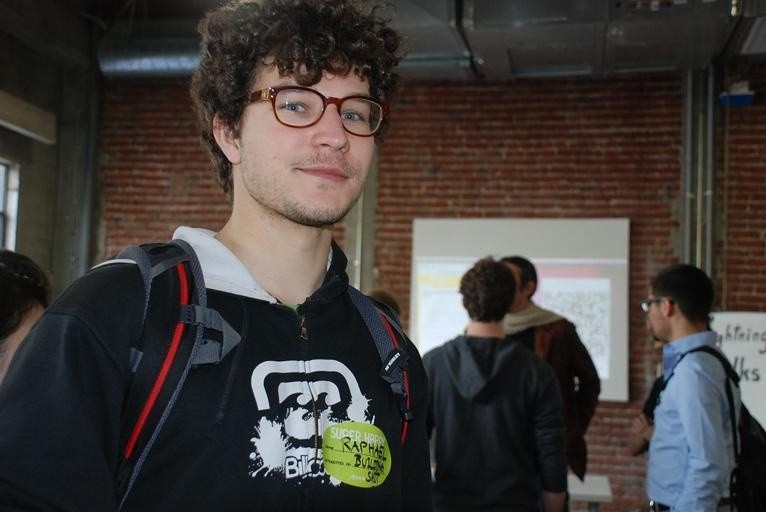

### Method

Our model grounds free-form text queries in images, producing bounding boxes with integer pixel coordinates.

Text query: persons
[0,246,49,386]
[497,256,599,511]
[421,256,569,512]
[641,263,741,512]
[0,0,438,512]
[628,316,711,456]
[362,289,402,317]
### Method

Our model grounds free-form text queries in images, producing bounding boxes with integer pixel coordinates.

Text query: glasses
[241,83,387,139]
[638,298,674,314]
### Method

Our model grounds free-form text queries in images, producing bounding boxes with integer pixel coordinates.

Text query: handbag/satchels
[726,396,764,511]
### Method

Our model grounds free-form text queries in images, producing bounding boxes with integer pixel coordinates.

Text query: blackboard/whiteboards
[707,311,766,433]
